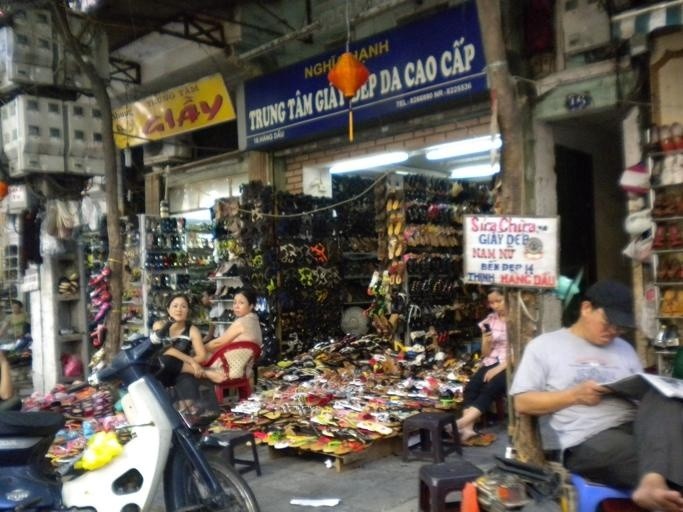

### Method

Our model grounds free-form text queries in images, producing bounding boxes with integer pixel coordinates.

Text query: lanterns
[326,52,370,143]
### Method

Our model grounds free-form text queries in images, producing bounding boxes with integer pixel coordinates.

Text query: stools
[205,430,262,477]
[566,472,630,511]
[399,411,484,511]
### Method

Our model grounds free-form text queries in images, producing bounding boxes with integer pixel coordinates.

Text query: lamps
[123,82,132,167]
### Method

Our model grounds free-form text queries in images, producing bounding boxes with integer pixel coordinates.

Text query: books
[494,453,550,483]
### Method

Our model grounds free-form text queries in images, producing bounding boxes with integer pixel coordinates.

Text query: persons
[437,285,516,446]
[0,351,24,414]
[190,287,260,381]
[507,277,681,510]
[0,297,27,346]
[198,284,225,343]
[149,292,228,389]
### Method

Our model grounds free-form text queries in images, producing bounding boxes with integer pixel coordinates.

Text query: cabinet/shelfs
[646,148,683,378]
[207,259,244,340]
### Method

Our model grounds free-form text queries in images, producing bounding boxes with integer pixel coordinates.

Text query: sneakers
[83,214,213,375]
[23,382,127,465]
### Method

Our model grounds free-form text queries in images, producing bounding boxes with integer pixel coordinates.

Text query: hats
[587,280,635,328]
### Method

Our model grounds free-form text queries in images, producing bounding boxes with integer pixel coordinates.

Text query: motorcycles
[0,314,263,512]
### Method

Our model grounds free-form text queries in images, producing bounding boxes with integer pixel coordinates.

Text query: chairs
[198,341,262,403]
